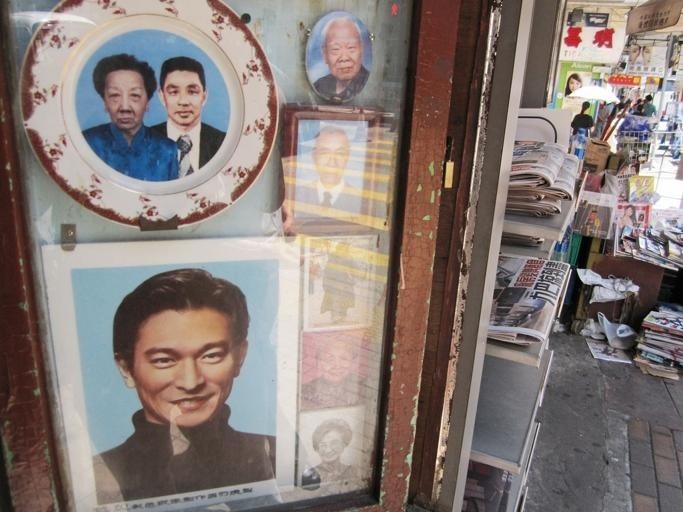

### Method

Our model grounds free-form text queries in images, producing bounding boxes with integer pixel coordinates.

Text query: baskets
[623,131,653,165]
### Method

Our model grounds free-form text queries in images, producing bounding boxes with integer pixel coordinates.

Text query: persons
[572,99,597,138]
[642,45,654,67]
[79,51,176,183]
[90,268,278,505]
[629,45,641,65]
[565,73,584,96]
[629,94,659,117]
[149,53,228,178]
[295,19,373,489]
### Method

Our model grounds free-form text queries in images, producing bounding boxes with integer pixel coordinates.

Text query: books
[614,175,683,382]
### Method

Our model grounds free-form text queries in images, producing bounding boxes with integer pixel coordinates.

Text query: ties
[176,136,193,179]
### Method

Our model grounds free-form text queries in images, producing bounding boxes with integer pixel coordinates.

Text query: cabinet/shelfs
[577,237,671,332]
[461,107,579,512]
